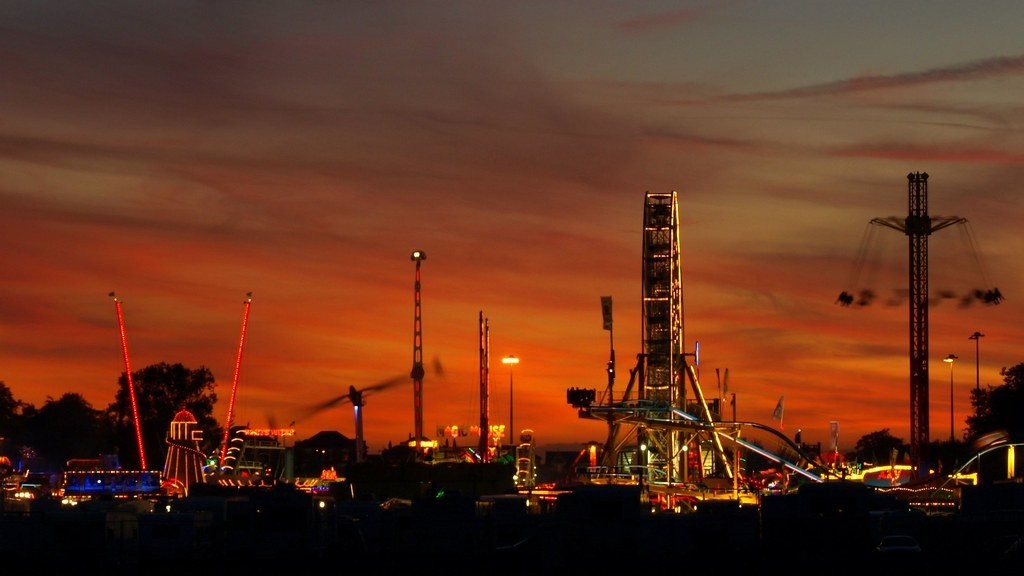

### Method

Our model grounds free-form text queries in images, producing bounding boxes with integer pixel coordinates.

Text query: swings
[837,216,1001,304]
